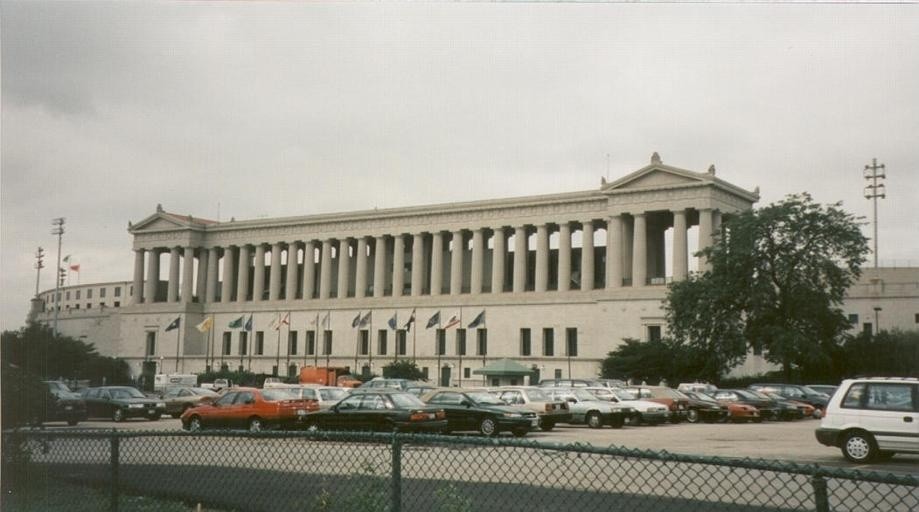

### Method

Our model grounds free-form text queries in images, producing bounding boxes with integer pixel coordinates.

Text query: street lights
[874,307,883,336]
[860,157,889,276]
[32,245,44,298]
[49,217,66,335]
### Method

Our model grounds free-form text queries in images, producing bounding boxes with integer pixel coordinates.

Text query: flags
[319,314,328,328]
[244,315,253,331]
[279,313,289,325]
[266,314,281,331]
[310,317,316,325]
[386,314,396,330]
[358,311,370,330]
[351,314,360,328]
[403,309,415,333]
[466,311,484,329]
[163,317,180,332]
[442,313,460,330]
[192,315,213,334]
[425,310,440,329]
[62,254,71,262]
[69,264,79,272]
[228,316,243,329]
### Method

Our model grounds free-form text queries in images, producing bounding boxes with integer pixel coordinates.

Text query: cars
[353,376,838,431]
[79,386,166,423]
[302,387,448,435]
[423,387,541,438]
[179,387,321,434]
[153,374,283,418]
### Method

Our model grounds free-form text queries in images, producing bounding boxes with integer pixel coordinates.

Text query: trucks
[298,366,362,389]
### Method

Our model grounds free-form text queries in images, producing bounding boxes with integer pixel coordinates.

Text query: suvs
[29,380,88,427]
[814,377,919,466]
